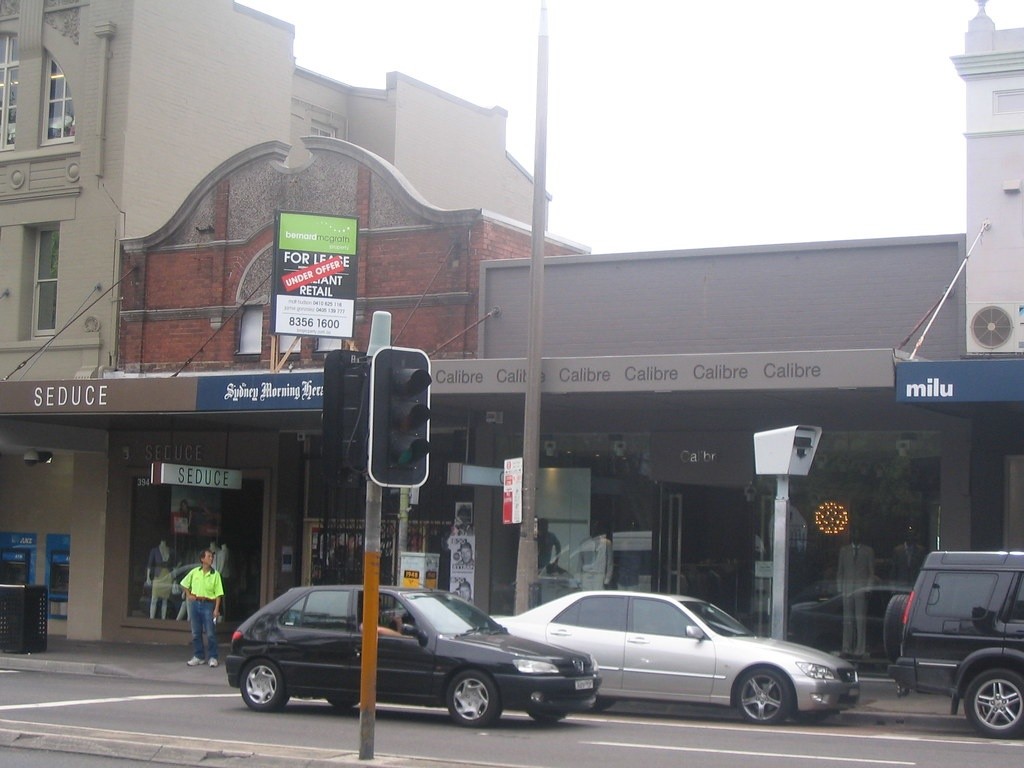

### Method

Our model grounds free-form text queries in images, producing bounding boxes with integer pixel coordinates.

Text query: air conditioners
[967,301,1024,352]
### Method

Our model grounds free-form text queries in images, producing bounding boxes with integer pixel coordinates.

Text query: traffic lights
[367,345,431,489]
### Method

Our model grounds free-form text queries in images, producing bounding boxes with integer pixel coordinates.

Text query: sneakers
[187,656,206,666]
[208,657,218,667]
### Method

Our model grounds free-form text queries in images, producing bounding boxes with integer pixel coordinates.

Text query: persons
[572,520,614,593]
[836,535,875,660]
[891,533,926,594]
[179,549,225,667]
[176,500,213,527]
[453,506,475,604]
[146,540,230,622]
[538,518,562,571]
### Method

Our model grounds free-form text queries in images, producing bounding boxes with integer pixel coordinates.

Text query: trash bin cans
[0,584,48,652]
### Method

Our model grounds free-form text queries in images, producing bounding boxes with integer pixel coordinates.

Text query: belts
[195,597,214,603]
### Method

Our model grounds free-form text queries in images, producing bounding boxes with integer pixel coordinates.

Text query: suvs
[881,549,1024,739]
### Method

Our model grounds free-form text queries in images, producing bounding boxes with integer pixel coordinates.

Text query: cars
[486,589,859,727]
[224,583,604,729]
